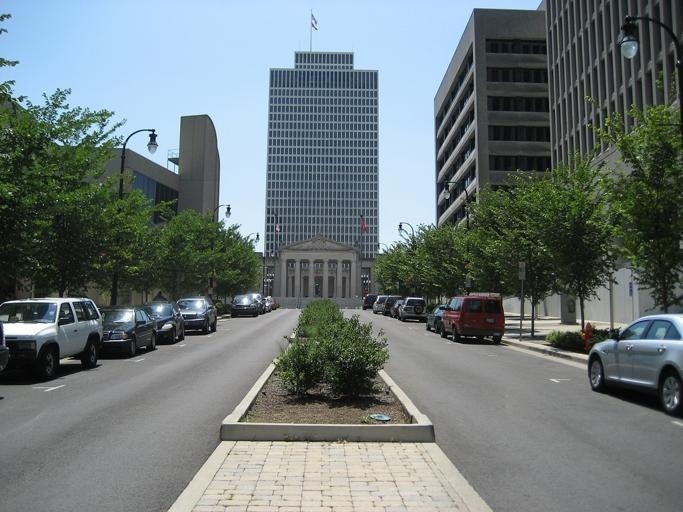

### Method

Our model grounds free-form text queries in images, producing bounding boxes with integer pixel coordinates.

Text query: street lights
[110,127,158,306]
[378,242,389,253]
[397,221,415,238]
[616,10,683,119]
[442,180,470,252]
[244,231,261,243]
[207,203,232,295]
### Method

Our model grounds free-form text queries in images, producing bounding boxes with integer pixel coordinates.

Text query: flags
[311,15,318,31]
[360,217,366,232]
[273,216,281,233]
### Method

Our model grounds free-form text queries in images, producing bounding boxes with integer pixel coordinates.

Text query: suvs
[381,295,402,316]
[397,296,427,323]
[0,296,105,380]
[175,294,218,335]
[438,295,507,345]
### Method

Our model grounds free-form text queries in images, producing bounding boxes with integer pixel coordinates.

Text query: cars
[97,304,159,358]
[586,312,683,413]
[141,299,186,344]
[371,294,387,314]
[0,319,11,375]
[361,293,377,311]
[392,299,403,318]
[249,294,277,314]
[425,302,447,334]
[229,294,258,318]
